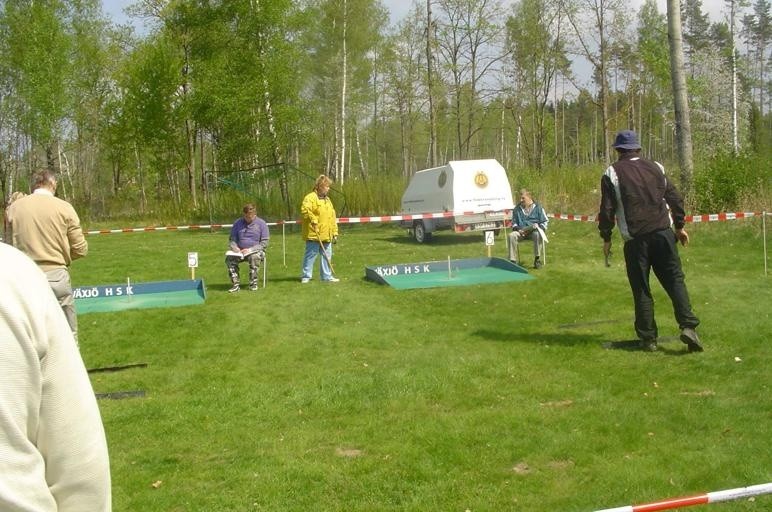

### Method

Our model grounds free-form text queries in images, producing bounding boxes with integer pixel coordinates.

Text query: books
[225,248,260,259]
[522,222,550,243]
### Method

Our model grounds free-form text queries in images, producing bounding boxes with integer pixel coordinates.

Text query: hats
[611,130,643,152]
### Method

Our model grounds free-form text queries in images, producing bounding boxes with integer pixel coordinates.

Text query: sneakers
[680,328,704,352]
[534,259,542,269]
[249,284,258,291]
[302,277,309,284]
[228,284,240,293]
[639,338,656,352]
[320,275,340,282]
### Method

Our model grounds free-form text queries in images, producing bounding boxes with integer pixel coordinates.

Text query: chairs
[518,238,546,266]
[261,252,266,288]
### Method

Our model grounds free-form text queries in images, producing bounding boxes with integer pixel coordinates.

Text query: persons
[0,242,114,512]
[508,188,550,269]
[595,131,704,355]
[299,175,341,285]
[223,201,270,291]
[3,191,30,247]
[6,167,88,353]
